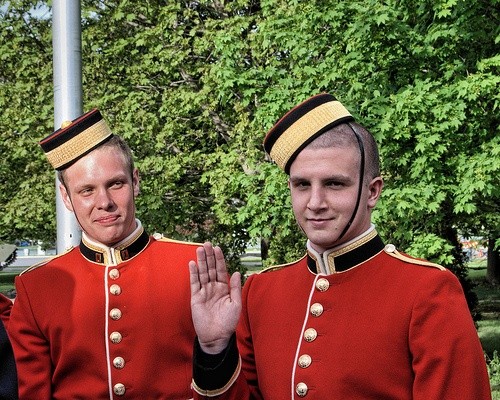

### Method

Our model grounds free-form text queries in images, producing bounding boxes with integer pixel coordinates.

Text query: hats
[263,94,355,176]
[38,107,114,172]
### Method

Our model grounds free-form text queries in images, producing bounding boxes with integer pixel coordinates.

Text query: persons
[186,93,492,400]
[4,108,232,399]
[0,293,23,400]
